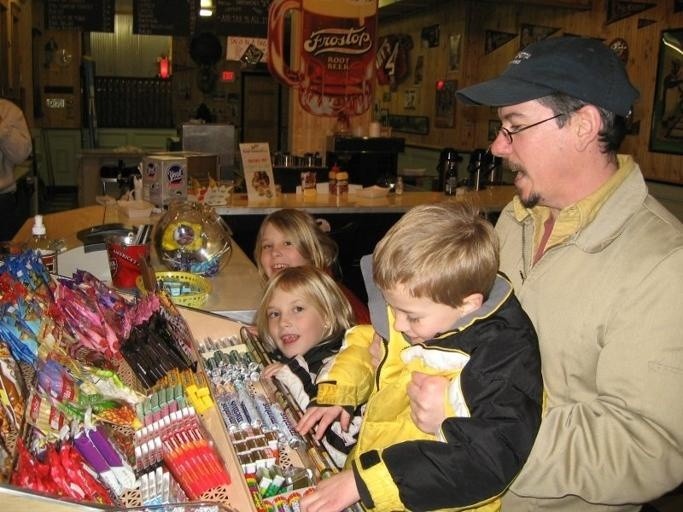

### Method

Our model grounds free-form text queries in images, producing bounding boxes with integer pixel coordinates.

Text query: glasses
[491,101,591,144]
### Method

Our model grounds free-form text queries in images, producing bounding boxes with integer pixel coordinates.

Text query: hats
[454,31,636,116]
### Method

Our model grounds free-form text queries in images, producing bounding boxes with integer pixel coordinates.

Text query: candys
[159,241,232,278]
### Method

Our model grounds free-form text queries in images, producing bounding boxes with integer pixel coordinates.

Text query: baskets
[136,271,211,307]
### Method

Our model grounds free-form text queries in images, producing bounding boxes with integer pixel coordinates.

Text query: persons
[252,207,370,326]
[0,98,34,245]
[254,265,372,470]
[368,34,682,512]
[294,196,550,512]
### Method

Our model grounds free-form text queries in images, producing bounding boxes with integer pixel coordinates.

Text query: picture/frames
[604,0,657,26]
[390,115,429,135]
[435,80,458,129]
[649,28,683,155]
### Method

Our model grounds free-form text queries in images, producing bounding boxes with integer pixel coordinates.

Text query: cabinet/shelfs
[2,0,81,131]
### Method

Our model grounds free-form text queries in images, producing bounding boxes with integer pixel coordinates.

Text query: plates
[356,185,390,198]
[122,200,153,219]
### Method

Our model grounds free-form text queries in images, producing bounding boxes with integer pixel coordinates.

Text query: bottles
[269,151,324,167]
[395,177,404,195]
[328,163,349,197]
[155,201,234,278]
[444,158,458,196]
[467,148,503,192]
[31,214,50,250]
[435,147,464,191]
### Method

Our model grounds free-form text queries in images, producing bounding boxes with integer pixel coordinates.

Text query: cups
[104,232,152,287]
[265,0,382,120]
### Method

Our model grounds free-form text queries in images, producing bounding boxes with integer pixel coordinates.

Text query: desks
[74,149,170,206]
